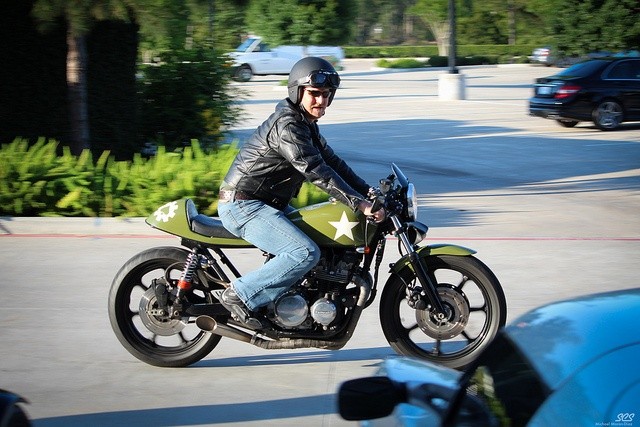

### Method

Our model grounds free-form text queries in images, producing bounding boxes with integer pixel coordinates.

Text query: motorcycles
[109,162,507,371]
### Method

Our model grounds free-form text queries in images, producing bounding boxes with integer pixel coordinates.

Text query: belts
[215,189,254,203]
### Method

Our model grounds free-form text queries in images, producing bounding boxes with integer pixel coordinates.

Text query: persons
[217,56,385,332]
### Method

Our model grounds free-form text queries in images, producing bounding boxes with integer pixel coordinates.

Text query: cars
[532,49,554,66]
[336,288,636,426]
[529,57,640,131]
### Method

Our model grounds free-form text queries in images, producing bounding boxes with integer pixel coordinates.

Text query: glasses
[289,69,340,89]
[307,88,332,97]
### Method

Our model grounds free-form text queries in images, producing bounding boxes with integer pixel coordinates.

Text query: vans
[217,37,344,82]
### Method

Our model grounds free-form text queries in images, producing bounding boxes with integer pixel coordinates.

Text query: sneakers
[219,285,273,331]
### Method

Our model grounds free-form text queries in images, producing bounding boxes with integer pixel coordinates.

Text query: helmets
[288,57,340,106]
